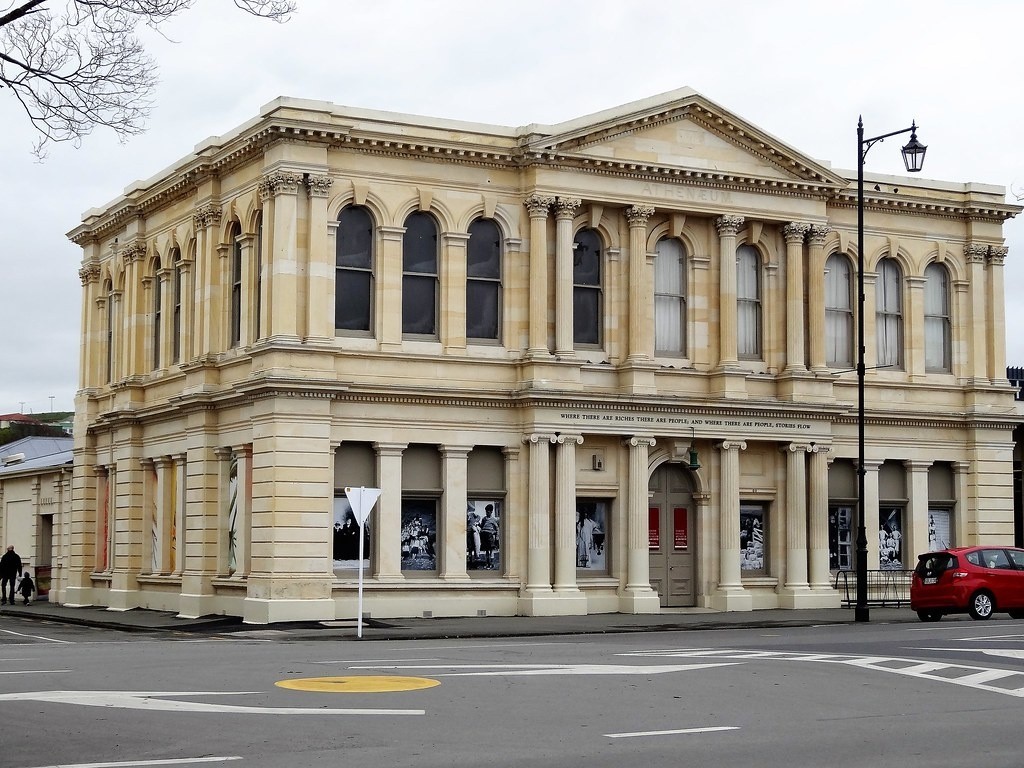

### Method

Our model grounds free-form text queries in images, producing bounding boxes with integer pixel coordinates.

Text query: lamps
[688,425,701,471]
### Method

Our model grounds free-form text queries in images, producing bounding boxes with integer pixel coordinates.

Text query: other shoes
[1,601,7,606]
[26,603,30,607]
[484,564,494,570]
[10,602,15,605]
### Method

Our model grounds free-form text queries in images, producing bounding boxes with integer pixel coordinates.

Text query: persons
[928,516,940,552]
[468,504,501,570]
[333,518,369,561]
[576,511,597,569]
[17,572,35,606]
[0,545,22,605]
[879,526,902,563]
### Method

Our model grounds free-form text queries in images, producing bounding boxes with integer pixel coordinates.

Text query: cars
[906,543,1024,622]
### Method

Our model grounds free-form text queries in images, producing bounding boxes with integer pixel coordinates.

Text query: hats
[24,572,30,577]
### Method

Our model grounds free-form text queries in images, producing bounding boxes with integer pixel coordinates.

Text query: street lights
[852,105,930,626]
